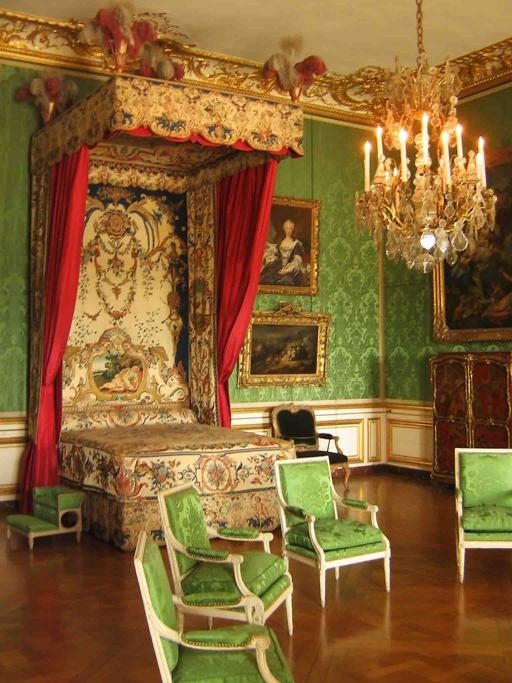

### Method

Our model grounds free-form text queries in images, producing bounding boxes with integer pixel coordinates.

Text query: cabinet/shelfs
[426,352,512,495]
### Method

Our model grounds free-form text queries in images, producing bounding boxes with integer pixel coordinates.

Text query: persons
[99,365,140,393]
[278,220,305,285]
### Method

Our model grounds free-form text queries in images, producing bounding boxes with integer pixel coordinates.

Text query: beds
[30,75,304,554]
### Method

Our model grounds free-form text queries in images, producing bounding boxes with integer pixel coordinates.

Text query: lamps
[354,1,498,274]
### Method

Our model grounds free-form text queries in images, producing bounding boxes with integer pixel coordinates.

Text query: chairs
[271,404,351,492]
[134,531,296,683]
[157,482,294,636]
[454,447,512,584]
[274,456,391,608]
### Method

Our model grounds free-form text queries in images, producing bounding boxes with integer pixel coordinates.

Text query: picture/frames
[256,196,321,295]
[432,75,512,343]
[236,303,330,388]
